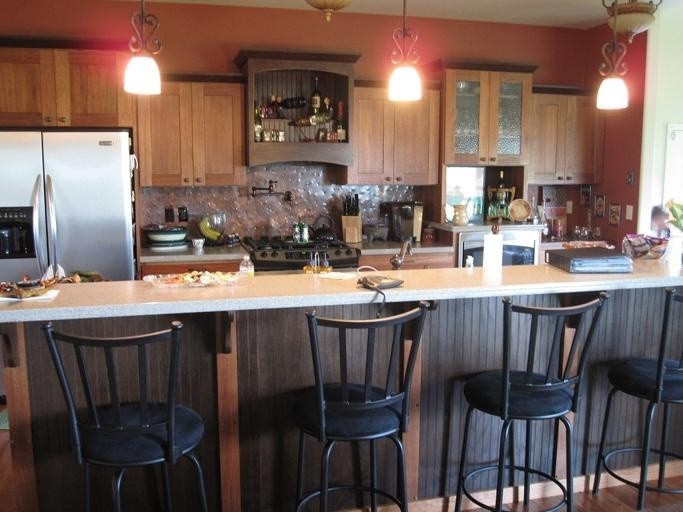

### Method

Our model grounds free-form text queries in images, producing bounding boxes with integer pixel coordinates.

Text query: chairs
[454,292,610,511]
[593,287,683,512]
[295,301,430,512]
[41,320,208,511]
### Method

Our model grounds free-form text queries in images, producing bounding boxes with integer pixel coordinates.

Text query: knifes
[342,193,359,215]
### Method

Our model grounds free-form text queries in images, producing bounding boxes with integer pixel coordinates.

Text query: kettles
[235,232,239,245]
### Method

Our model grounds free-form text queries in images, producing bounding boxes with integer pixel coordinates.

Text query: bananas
[197,217,221,241]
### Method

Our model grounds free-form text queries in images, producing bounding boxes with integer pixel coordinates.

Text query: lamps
[304,0,351,23]
[123,0,163,96]
[385,1,425,103]
[602,0,662,42]
[596,0,630,109]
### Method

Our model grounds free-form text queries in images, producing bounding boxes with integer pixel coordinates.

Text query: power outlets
[178,207,187,221]
[164,208,174,221]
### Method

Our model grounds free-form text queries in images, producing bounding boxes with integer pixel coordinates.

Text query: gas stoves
[240,236,362,270]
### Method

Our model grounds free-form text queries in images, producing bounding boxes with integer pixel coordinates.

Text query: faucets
[390,239,413,270]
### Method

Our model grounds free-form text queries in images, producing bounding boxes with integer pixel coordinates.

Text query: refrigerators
[0,127,138,280]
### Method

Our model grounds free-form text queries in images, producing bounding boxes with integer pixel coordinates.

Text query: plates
[507,199,531,222]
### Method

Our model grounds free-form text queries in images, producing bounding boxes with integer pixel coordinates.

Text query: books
[545,247,633,273]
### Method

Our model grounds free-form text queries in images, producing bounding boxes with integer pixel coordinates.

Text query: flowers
[665,198,683,232]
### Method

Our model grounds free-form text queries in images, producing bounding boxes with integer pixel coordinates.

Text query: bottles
[539,198,551,242]
[254,100,262,142]
[497,170,506,207]
[239,254,254,279]
[531,195,540,226]
[228,235,233,248]
[334,100,347,142]
[451,203,470,226]
[310,75,321,115]
[232,234,236,246]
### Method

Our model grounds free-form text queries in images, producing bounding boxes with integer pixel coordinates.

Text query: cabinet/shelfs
[233,49,362,168]
[140,254,243,278]
[525,93,605,186]
[0,47,137,126]
[336,87,440,185]
[441,68,532,167]
[358,247,455,269]
[138,82,246,188]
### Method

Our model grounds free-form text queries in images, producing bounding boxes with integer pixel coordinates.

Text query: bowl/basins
[142,225,187,242]
[149,240,188,253]
[191,239,205,248]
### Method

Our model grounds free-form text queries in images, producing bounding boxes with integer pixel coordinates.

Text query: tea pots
[308,214,337,242]
